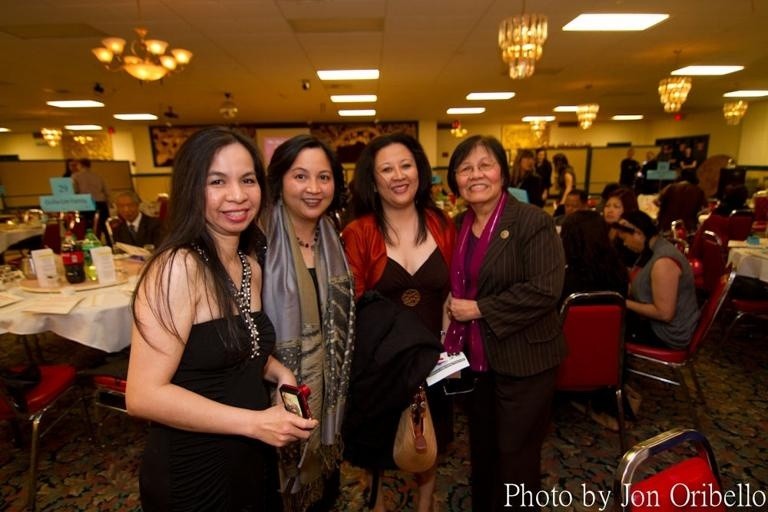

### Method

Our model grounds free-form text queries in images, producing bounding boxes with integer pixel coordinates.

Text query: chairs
[0,357,92,512]
[554,190,768,512]
[78,352,129,448]
[154,194,168,219]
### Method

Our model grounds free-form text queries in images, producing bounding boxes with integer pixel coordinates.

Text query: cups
[435,201,444,210]
[31,247,58,287]
[22,257,36,280]
[90,246,115,282]
[127,276,140,289]
[60,286,76,296]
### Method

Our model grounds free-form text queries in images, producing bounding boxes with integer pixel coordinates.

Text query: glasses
[455,161,497,177]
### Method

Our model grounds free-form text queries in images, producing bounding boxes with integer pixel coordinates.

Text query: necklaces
[295,225,322,251]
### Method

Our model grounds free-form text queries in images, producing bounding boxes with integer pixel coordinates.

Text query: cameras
[279,383,312,420]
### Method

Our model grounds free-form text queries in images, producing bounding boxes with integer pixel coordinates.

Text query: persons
[603,187,638,226]
[125,127,320,512]
[70,158,109,240]
[564,190,589,214]
[610,208,703,351]
[508,146,574,211]
[339,133,456,512]
[613,138,767,243]
[553,209,629,306]
[63,159,77,177]
[236,135,356,511]
[104,189,165,251]
[444,134,570,512]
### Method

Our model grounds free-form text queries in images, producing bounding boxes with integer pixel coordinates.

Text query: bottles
[447,192,457,210]
[62,231,85,284]
[81,229,103,281]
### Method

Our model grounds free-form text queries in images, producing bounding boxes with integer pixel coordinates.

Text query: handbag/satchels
[570,379,642,432]
[393,386,438,474]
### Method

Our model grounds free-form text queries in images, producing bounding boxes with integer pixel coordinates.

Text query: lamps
[74,136,95,147]
[575,102,600,131]
[530,121,547,143]
[723,99,750,126]
[91,0,193,84]
[657,49,692,114]
[450,122,468,138]
[41,127,62,147]
[497,0,549,80]
